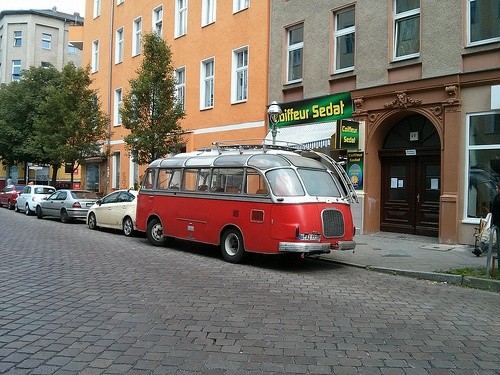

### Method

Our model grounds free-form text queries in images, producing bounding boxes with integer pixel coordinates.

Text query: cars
[14,185,57,215]
[0,184,23,210]
[35,188,100,223]
[86,189,138,237]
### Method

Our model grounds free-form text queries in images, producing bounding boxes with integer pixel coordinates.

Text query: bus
[136,138,360,263]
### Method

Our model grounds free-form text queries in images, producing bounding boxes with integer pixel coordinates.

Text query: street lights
[266,101,283,144]
